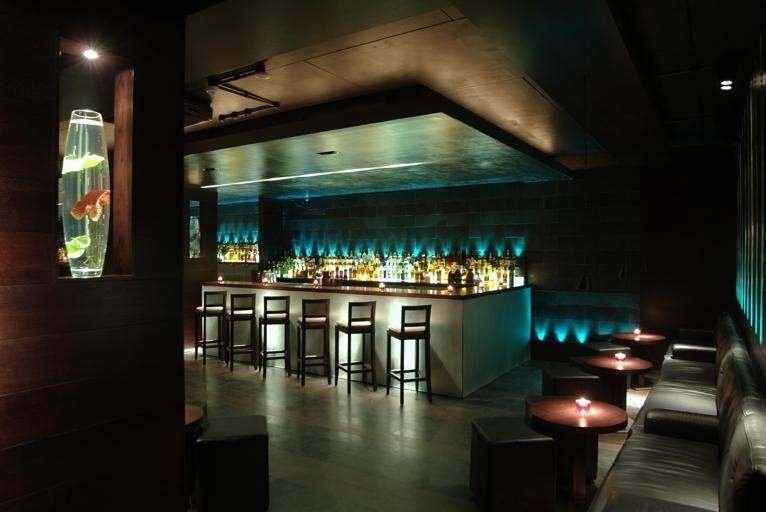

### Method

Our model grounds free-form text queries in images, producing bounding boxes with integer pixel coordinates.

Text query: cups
[575,398,592,412]
[614,353,627,364]
[634,329,641,337]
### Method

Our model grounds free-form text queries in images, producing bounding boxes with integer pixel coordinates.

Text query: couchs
[585,299,765,512]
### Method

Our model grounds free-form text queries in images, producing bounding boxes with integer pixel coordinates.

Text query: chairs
[189,291,433,407]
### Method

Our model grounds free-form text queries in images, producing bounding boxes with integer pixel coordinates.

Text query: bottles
[190,216,202,257]
[211,238,527,295]
[62,109,111,280]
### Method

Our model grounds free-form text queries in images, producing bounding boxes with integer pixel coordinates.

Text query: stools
[471,416,552,504]
[194,411,270,510]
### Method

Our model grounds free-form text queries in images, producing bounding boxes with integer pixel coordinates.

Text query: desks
[527,331,669,506]
